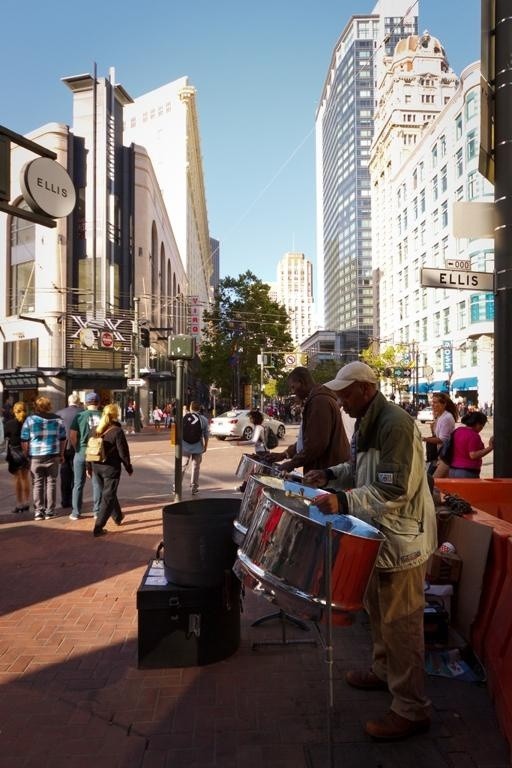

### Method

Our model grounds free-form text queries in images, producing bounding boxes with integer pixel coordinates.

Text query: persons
[232,394,303,489]
[0,393,132,538]
[181,400,209,495]
[388,392,492,478]
[303,361,437,739]
[125,401,176,434]
[262,366,351,480]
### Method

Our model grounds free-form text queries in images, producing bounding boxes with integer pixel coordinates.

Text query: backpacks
[85,426,121,463]
[259,424,278,449]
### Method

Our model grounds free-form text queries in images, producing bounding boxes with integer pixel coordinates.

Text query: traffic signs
[127,378,147,387]
[421,259,495,290]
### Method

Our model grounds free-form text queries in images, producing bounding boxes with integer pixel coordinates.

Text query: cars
[209,409,286,442]
[417,406,435,424]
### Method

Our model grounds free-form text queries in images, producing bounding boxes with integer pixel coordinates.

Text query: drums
[236,455,303,483]
[232,490,387,627]
[234,474,332,547]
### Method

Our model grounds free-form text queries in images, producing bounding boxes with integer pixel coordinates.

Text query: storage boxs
[418,601,450,655]
[133,553,249,670]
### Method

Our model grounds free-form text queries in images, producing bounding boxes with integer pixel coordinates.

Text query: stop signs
[100,332,114,348]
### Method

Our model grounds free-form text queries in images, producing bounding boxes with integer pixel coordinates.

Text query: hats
[322,361,377,391]
[86,392,99,403]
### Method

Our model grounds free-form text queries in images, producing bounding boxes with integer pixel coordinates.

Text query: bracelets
[283,450,289,459]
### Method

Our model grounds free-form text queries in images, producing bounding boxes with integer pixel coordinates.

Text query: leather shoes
[362,709,431,741]
[346,666,389,691]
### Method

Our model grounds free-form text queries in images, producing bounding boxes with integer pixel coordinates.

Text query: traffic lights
[124,364,133,378]
[211,389,218,396]
[140,328,150,348]
[304,354,309,366]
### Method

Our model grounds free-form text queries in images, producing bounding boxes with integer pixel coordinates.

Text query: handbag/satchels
[183,413,202,444]
[438,434,453,465]
[9,446,26,465]
